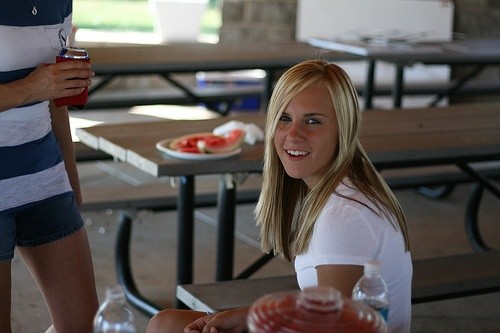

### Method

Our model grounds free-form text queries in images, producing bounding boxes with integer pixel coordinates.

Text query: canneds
[58,46,89,60]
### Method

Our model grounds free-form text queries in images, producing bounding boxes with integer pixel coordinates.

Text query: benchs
[355,79,500,95]
[78,162,500,213]
[176,251,500,312]
[81,84,261,106]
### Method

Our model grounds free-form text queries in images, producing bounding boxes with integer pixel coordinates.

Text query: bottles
[352,259,390,323]
[92,284,137,333]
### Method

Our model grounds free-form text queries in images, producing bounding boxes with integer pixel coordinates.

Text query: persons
[0,0,101,333]
[145,60,411,333]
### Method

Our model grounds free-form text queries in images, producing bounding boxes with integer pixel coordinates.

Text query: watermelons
[177,127,245,153]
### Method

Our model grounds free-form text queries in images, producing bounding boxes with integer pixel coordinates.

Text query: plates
[156,136,242,160]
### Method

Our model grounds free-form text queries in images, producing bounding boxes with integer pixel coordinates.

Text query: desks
[85,33,500,116]
[78,104,499,317]
